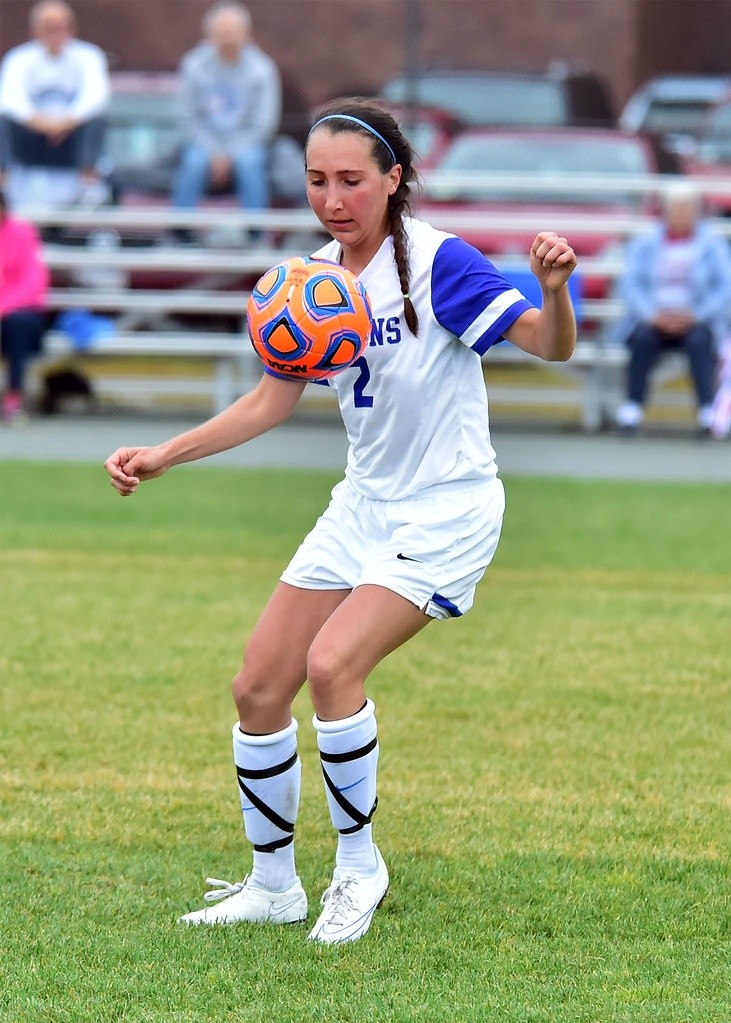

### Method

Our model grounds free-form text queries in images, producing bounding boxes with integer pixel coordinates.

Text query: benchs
[10,328,729,436]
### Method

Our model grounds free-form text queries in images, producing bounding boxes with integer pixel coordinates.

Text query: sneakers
[305,843,389,944]
[179,869,308,925]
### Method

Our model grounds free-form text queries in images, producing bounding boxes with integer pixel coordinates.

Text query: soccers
[247,257,369,378]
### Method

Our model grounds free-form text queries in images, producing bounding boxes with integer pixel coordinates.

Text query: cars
[87,42,730,334]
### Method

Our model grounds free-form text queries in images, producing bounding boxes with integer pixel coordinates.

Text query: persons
[0,0,111,213]
[102,100,578,944]
[168,0,283,248]
[0,192,50,418]
[611,186,731,434]
